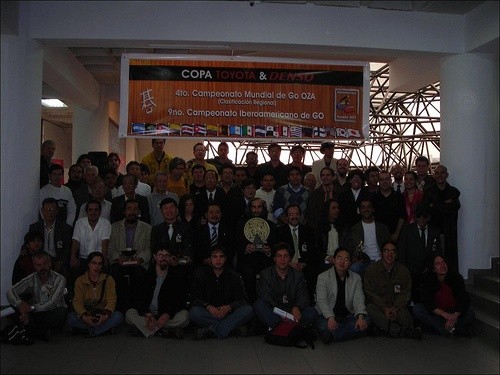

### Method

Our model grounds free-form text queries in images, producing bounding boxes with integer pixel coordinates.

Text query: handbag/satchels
[264,316,315,350]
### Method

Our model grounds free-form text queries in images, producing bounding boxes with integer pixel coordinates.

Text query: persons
[6,138,470,344]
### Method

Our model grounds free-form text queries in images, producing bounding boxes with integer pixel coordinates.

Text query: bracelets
[31,306,34,310]
[357,315,365,320]
[208,305,212,311]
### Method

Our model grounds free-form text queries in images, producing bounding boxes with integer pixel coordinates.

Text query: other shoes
[69,327,87,336]
[195,327,217,340]
[32,327,51,343]
[322,331,333,344]
[423,326,440,336]
[103,327,114,335]
[453,326,472,338]
[129,326,145,337]
[160,327,185,340]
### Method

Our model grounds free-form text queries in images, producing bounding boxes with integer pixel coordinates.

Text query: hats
[414,201,431,215]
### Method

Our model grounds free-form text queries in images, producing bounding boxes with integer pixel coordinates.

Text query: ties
[397,185,401,192]
[209,192,213,200]
[326,191,331,201]
[168,224,174,240]
[210,226,217,248]
[420,228,426,248]
[291,228,298,263]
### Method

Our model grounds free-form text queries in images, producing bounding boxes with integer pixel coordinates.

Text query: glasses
[414,214,430,219]
[336,257,351,261]
[156,253,170,259]
